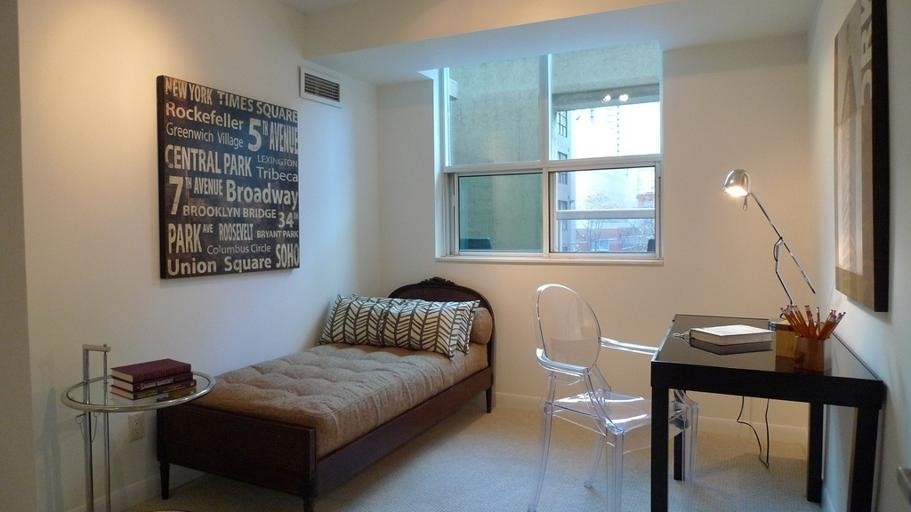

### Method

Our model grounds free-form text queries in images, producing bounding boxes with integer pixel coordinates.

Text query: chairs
[528,283,698,512]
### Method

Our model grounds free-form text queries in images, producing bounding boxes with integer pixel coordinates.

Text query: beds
[154,277,496,512]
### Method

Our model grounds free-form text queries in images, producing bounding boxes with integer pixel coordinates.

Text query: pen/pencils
[780,304,846,337]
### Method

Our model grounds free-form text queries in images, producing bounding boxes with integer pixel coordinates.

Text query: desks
[60,342,216,512]
[648,314,887,511]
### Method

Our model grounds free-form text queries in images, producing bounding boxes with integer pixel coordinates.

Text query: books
[689,337,776,356]
[110,387,197,409]
[689,323,776,346]
[109,358,196,401]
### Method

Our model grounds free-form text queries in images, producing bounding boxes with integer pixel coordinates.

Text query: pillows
[321,296,466,359]
[349,293,483,355]
[471,307,493,344]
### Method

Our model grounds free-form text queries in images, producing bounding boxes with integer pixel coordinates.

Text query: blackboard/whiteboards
[156,75,300,277]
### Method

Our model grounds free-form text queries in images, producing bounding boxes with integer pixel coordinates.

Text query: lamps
[719,171,818,316]
